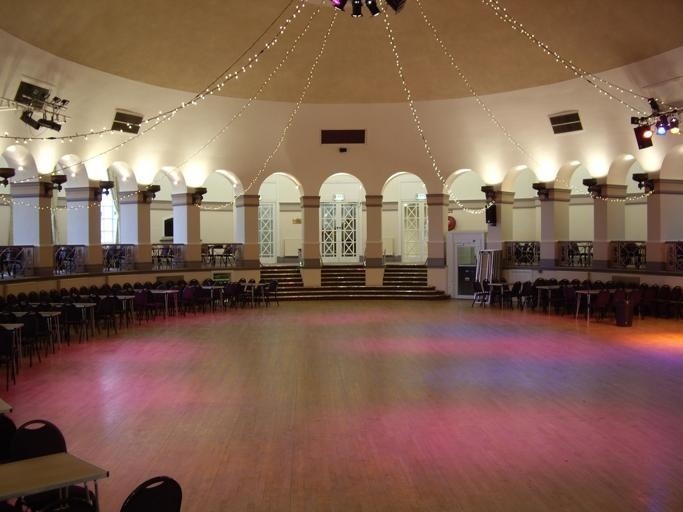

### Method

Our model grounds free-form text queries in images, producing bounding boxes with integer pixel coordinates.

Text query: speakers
[634,124,653,150]
[485,205,497,224]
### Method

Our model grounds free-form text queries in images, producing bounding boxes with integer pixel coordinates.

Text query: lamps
[631,97,678,138]
[332,0,406,18]
[480,173,654,200]
[0,168,15,187]
[192,188,207,204]
[143,185,160,202]
[46,175,67,193]
[95,180,114,195]
[19,94,70,131]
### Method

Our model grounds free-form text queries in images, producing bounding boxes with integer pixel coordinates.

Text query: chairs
[472,243,683,322]
